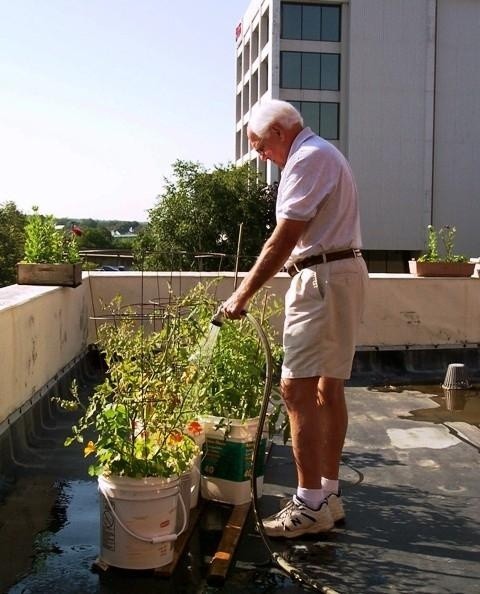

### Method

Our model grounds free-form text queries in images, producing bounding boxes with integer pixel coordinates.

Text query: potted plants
[409,222,476,277]
[189,291,283,506]
[116,306,207,512]
[71,348,191,569]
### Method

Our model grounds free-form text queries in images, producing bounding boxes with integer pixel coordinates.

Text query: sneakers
[254,489,346,538]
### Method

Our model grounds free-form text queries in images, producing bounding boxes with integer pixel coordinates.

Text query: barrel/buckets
[441,363,473,389]
[443,389,471,412]
[97,413,270,570]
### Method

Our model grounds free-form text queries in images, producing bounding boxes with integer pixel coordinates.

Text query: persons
[223,100,368,538]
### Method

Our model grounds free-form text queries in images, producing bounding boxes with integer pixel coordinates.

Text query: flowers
[23,203,83,265]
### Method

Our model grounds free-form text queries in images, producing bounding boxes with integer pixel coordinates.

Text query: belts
[287,248,362,277]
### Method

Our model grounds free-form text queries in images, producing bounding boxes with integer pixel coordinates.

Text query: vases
[16,262,82,287]
